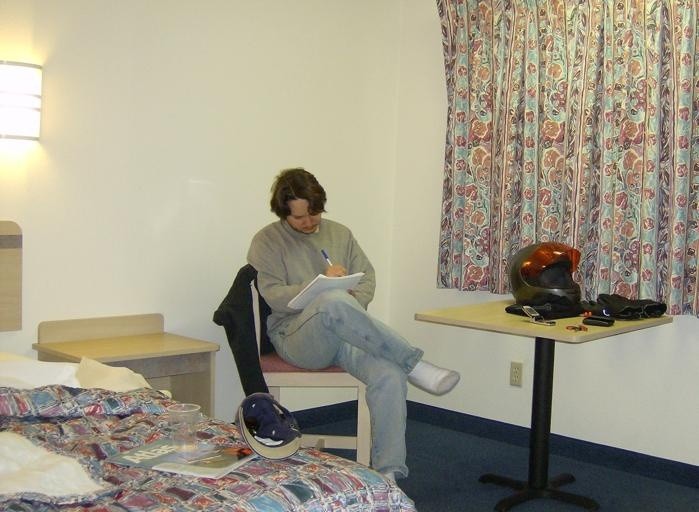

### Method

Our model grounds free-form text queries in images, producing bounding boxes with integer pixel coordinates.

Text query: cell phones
[521,303,557,326]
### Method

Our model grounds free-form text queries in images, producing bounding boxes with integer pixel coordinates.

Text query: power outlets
[510,362,522,386]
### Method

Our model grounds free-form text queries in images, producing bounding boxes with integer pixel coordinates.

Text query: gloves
[597,293,667,319]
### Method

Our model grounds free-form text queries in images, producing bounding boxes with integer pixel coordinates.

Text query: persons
[245,168,461,486]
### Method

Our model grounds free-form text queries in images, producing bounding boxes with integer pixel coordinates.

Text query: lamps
[0,60,42,141]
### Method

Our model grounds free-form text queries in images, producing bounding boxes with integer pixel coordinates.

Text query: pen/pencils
[320,249,332,267]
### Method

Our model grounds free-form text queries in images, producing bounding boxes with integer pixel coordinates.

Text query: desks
[414,300,673,512]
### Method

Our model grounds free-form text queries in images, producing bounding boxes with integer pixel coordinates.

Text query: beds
[0,220,419,512]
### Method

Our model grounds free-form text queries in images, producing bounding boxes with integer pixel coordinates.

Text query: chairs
[245,264,371,466]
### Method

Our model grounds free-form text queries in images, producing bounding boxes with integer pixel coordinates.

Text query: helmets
[510,241,582,304]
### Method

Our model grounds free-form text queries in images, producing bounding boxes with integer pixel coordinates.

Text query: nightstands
[30,312,220,418]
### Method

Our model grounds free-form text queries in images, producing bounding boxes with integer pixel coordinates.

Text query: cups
[168,404,201,455]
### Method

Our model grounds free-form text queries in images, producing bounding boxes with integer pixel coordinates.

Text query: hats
[238,392,301,461]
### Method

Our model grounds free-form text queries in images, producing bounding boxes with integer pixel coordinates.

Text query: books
[284,271,366,311]
[104,435,259,480]
